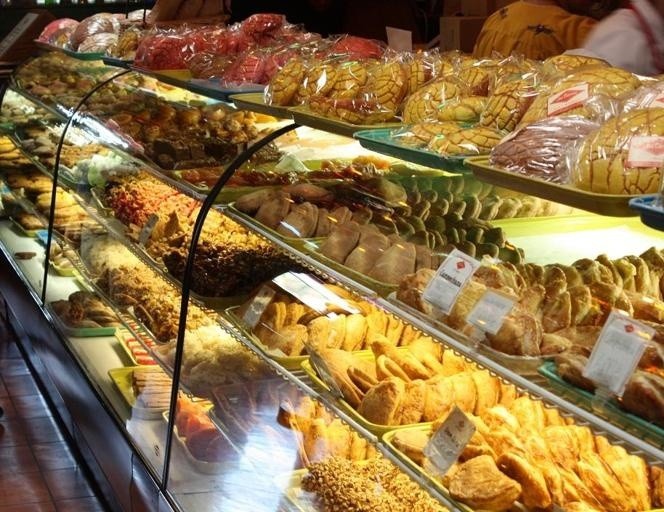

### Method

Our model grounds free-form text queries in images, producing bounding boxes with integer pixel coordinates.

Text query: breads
[329,61,367,100]
[308,96,374,124]
[236,279,418,352]
[43,7,147,58]
[293,62,338,105]
[489,114,595,182]
[489,61,521,96]
[620,76,664,110]
[555,341,664,428]
[401,119,459,149]
[436,94,488,119]
[544,53,610,75]
[364,62,407,121]
[404,56,436,93]
[516,67,639,132]
[266,59,302,107]
[457,66,489,98]
[236,164,572,284]
[397,245,664,354]
[570,106,664,196]
[429,128,498,161]
[402,79,459,124]
[394,397,664,512]
[479,81,533,131]
[309,338,518,425]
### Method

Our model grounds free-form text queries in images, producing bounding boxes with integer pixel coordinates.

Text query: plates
[163,266,250,310]
[209,400,297,476]
[382,424,494,512]
[108,365,173,420]
[42,250,80,276]
[33,39,106,61]
[186,78,267,103]
[162,409,241,475]
[72,273,92,293]
[15,129,39,150]
[48,299,116,338]
[230,92,402,141]
[132,239,170,268]
[173,157,354,203]
[36,230,56,248]
[126,301,178,345]
[90,276,134,310]
[227,201,592,252]
[152,345,211,398]
[387,289,559,377]
[276,467,322,512]
[302,216,664,299]
[74,246,103,278]
[100,55,134,70]
[629,194,664,231]
[537,360,664,444]
[464,156,655,218]
[299,349,436,442]
[89,187,116,217]
[223,304,410,369]
[353,127,470,176]
[10,215,46,237]
[114,329,146,365]
[155,69,198,88]
[53,167,93,192]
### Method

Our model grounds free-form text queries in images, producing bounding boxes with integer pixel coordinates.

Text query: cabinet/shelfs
[0,55,128,328]
[41,66,300,511]
[158,124,663,512]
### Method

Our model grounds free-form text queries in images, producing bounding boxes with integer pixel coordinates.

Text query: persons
[585,0,664,76]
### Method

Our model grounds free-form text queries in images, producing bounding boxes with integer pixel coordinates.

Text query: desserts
[0,51,305,470]
